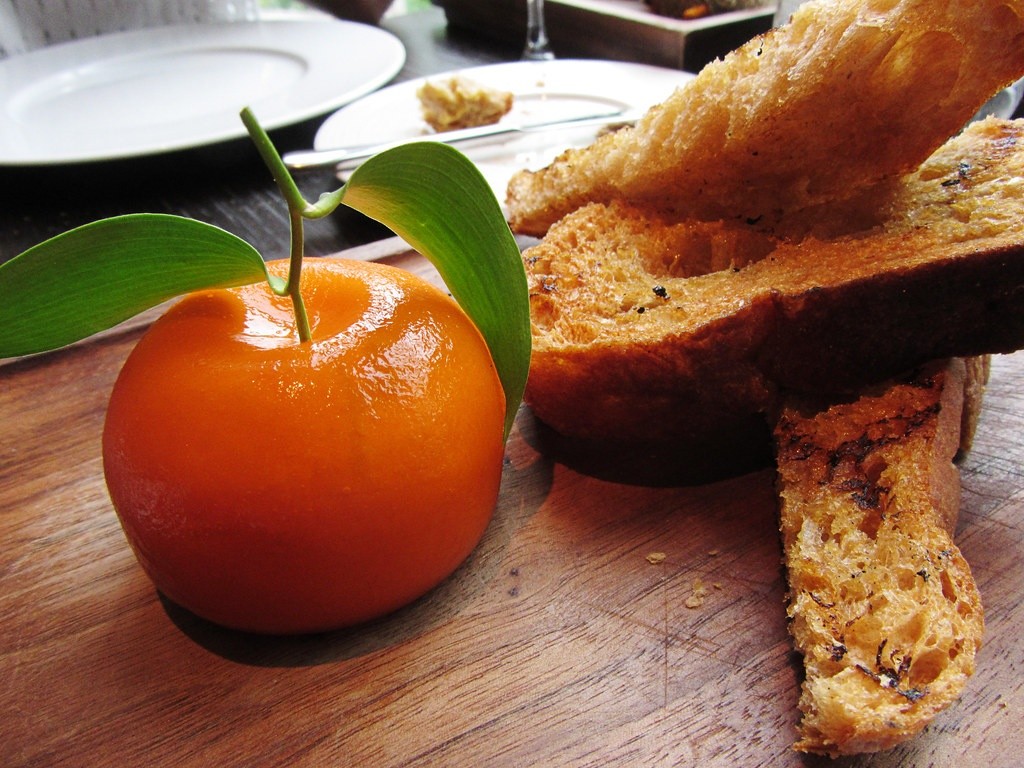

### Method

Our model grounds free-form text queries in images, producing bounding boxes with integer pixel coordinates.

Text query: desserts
[103,257,505,633]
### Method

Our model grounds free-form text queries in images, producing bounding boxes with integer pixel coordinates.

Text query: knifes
[281,107,621,167]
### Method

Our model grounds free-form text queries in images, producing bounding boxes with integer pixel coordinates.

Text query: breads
[417,74,515,132]
[500,0,1024,758]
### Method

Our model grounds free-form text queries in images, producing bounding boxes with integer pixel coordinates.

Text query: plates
[0,15,407,164]
[308,61,716,237]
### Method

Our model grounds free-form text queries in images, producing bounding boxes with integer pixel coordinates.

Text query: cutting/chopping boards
[1,201,1024,767]
